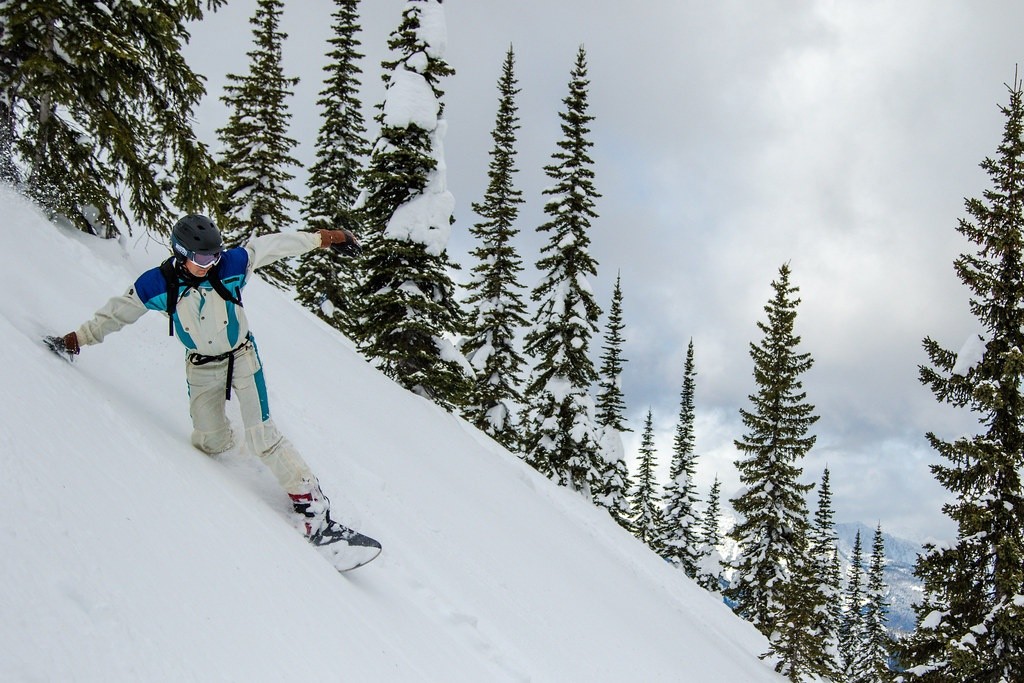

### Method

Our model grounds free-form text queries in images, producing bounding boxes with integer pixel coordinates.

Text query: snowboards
[285,504,385,575]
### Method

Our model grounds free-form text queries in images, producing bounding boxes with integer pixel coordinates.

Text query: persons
[40,214,356,542]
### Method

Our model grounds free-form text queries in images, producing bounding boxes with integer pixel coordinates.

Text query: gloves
[46,332,79,356]
[315,228,363,258]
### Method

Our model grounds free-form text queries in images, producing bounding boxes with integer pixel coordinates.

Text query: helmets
[171,215,225,264]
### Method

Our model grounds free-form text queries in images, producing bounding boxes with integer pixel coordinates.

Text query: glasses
[192,251,222,268]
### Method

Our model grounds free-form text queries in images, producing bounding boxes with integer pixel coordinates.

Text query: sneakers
[288,486,330,541]
